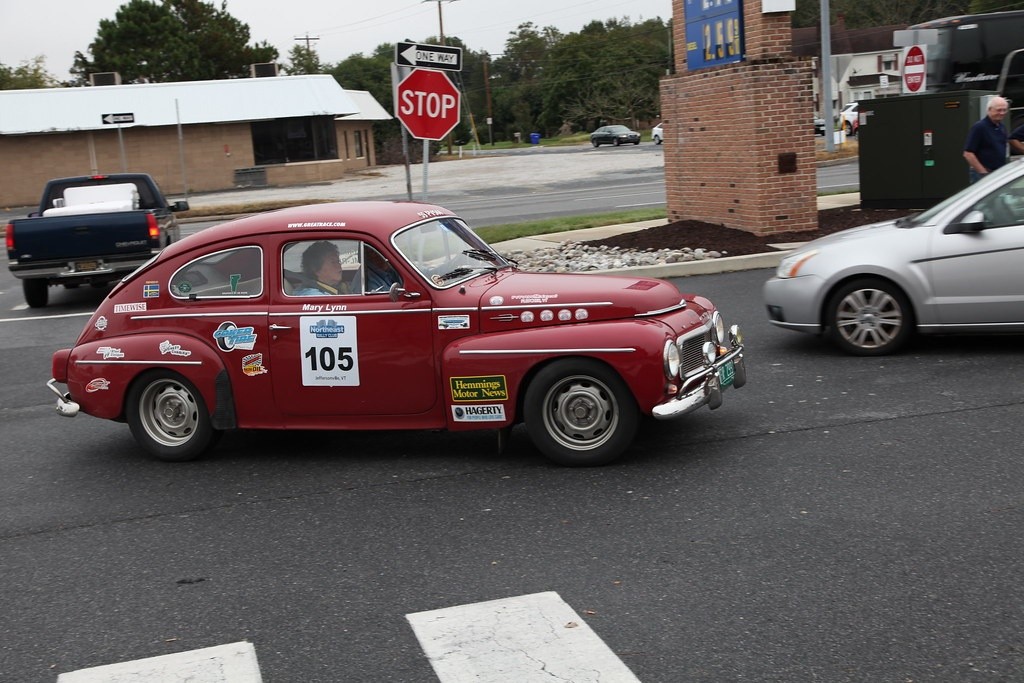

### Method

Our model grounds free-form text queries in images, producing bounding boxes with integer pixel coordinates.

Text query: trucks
[901,9,1023,151]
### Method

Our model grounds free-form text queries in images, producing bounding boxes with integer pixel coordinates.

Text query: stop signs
[396,68,463,143]
[901,44,926,92]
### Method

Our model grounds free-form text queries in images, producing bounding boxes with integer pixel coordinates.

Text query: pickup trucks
[6,173,190,309]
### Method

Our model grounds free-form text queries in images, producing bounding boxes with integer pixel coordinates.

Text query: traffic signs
[394,41,464,69]
[102,113,135,123]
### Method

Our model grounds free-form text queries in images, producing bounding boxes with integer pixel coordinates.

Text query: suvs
[837,101,858,134]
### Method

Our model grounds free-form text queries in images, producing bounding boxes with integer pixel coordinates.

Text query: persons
[350,242,447,294]
[1008,123,1024,153]
[961,97,1009,184]
[293,242,343,295]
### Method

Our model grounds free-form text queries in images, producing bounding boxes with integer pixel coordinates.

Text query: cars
[813,113,826,134]
[45,199,747,466]
[651,122,664,143]
[589,124,642,147]
[764,152,1023,352]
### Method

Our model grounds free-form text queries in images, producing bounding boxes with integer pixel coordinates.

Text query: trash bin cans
[234,167,251,186]
[530,133,540,144]
[250,166,267,185]
